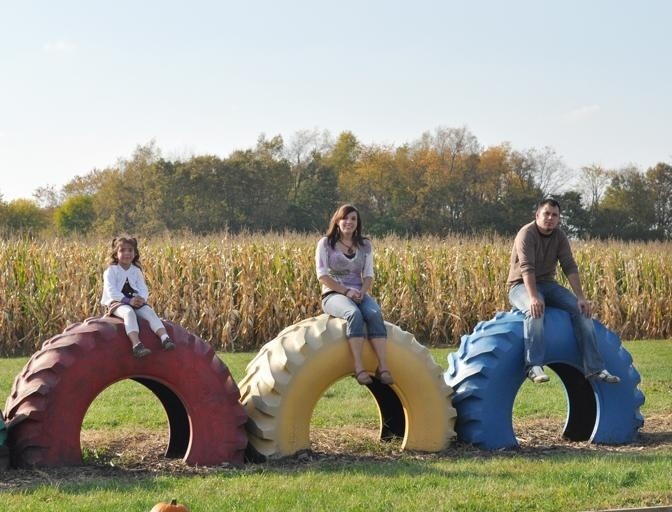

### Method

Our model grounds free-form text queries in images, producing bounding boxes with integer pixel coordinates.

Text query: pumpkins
[150,499,189,512]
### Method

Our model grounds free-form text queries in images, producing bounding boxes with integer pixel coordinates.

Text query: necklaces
[338,238,356,253]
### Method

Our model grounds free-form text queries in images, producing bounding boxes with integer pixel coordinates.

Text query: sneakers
[528,366,550,383]
[375,366,394,384]
[132,343,152,357]
[162,337,176,352]
[354,368,373,385]
[593,370,620,383]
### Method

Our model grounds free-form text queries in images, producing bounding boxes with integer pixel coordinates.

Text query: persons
[100,235,177,359]
[504,199,623,384]
[314,204,395,387]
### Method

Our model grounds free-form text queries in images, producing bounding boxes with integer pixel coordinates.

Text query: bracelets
[124,297,130,304]
[345,287,351,296]
